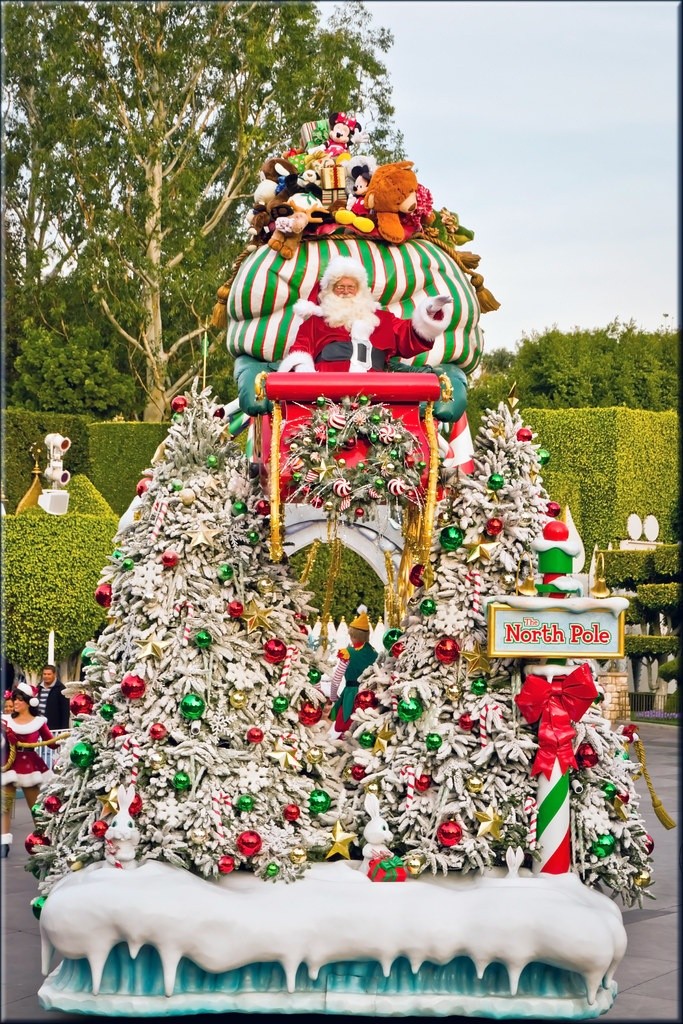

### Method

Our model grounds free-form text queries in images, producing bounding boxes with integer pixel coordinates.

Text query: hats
[17,682,40,707]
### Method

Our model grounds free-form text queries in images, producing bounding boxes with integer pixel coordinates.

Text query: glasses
[13,694,23,702]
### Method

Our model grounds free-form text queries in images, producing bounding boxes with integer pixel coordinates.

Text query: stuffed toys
[245,112,437,258]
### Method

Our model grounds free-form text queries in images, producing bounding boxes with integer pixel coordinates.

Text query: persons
[2,665,73,857]
[279,255,455,377]
[330,614,379,728]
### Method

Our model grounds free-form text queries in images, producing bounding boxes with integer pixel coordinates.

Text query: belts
[15,746,34,752]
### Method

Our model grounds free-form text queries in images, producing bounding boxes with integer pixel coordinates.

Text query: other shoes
[1,833,13,858]
